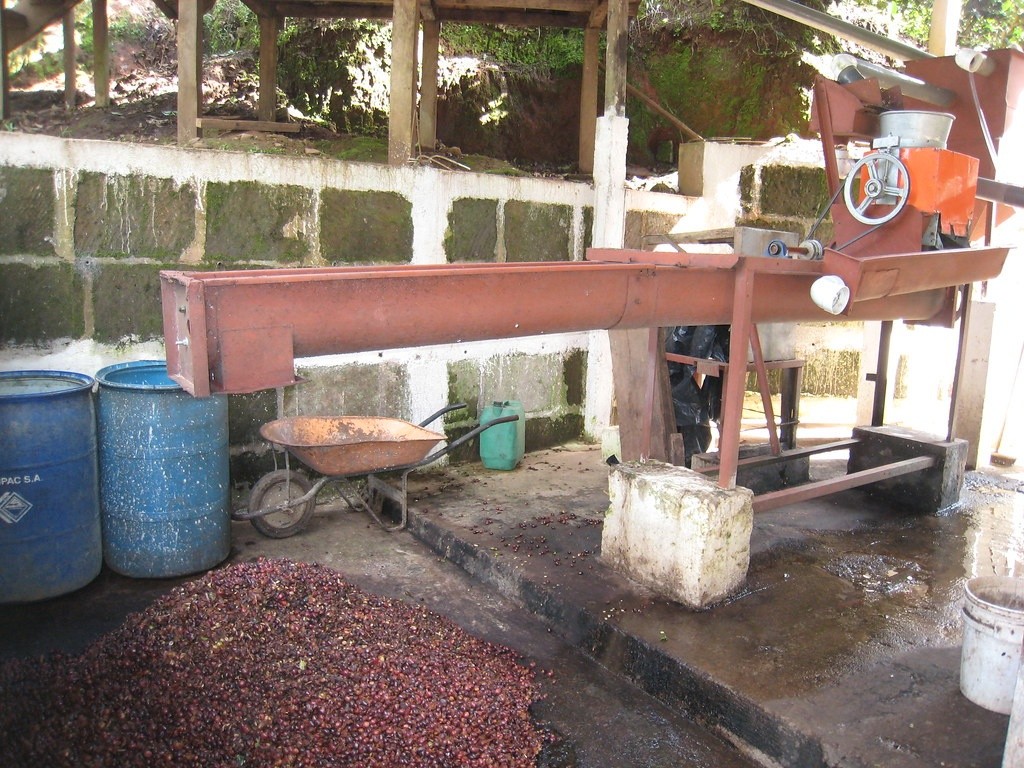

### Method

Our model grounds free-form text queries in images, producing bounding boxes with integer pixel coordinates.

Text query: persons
[664,322,731,469]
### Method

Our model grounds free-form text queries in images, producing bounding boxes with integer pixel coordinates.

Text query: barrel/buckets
[480,399,526,471]
[1,370,103,603]
[960,575,1024,715]
[96,360,231,577]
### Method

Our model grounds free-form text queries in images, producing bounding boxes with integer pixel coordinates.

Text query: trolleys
[263,401,522,535]
